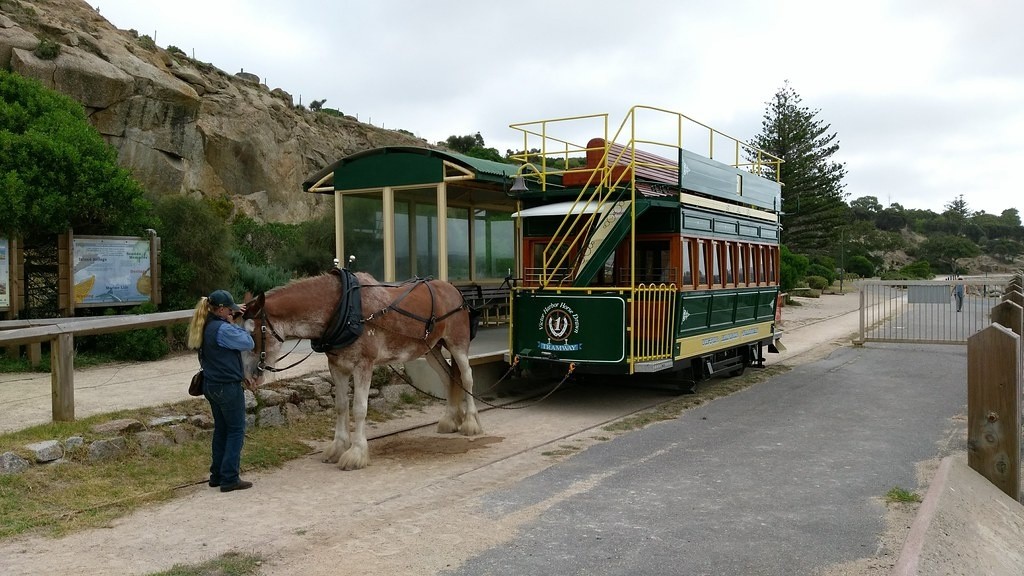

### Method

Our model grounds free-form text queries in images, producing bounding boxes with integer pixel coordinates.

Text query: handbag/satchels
[188,370,204,396]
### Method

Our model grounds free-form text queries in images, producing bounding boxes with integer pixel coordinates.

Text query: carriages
[189,106,787,471]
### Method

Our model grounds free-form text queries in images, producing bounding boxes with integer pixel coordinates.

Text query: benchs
[563,138,679,197]
[458,285,510,327]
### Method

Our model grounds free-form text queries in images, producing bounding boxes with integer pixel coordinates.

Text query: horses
[232,273,483,471]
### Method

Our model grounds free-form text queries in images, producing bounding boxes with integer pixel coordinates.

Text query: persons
[950,277,968,311]
[187,291,255,492]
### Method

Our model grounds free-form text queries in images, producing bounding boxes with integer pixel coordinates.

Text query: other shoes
[956,309,961,312]
[221,479,254,492]
[208,481,220,487]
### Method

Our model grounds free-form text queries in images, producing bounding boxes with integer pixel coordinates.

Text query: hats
[207,290,240,312]
[957,276,964,280]
